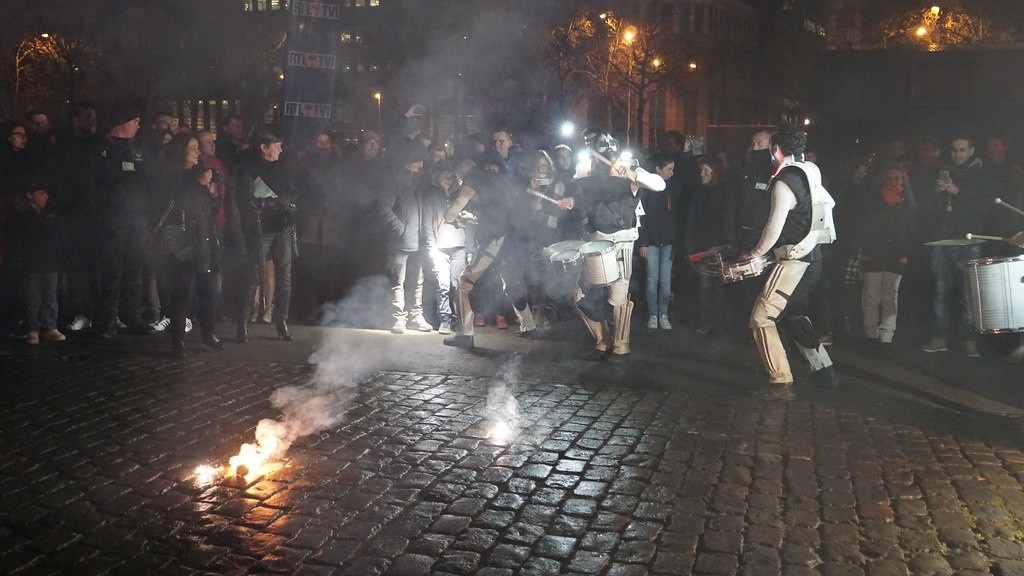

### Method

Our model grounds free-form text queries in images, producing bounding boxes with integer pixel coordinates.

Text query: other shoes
[173,344,186,356]
[130,324,157,335]
[100,327,117,338]
[184,317,193,332]
[1003,346,1024,362]
[66,315,92,330]
[237,327,249,343]
[580,348,609,361]
[387,307,556,349]
[819,336,833,345]
[203,334,226,349]
[794,366,840,388]
[276,322,293,340]
[608,351,632,366]
[153,317,171,331]
[748,382,796,401]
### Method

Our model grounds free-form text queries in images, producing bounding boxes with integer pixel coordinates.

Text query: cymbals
[530,248,558,260]
[924,238,986,246]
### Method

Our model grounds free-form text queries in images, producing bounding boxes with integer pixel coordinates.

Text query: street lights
[622,25,637,144]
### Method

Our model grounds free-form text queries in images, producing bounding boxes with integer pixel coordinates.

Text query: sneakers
[660,314,672,330]
[962,340,981,358]
[44,329,66,341]
[648,315,659,328]
[26,332,40,345]
[921,338,948,352]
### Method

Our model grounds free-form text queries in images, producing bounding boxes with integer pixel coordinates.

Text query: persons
[802,132,1024,362]
[738,127,839,399]
[557,139,666,364]
[0,102,584,356]
[630,130,778,335]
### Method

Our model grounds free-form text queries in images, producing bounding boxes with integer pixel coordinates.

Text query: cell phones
[939,170,949,184]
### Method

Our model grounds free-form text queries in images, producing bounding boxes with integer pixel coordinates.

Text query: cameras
[209,168,224,184]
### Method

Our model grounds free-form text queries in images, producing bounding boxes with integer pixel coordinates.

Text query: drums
[961,253,1024,334]
[550,249,583,276]
[717,250,777,283]
[581,239,622,288]
[545,239,589,259]
